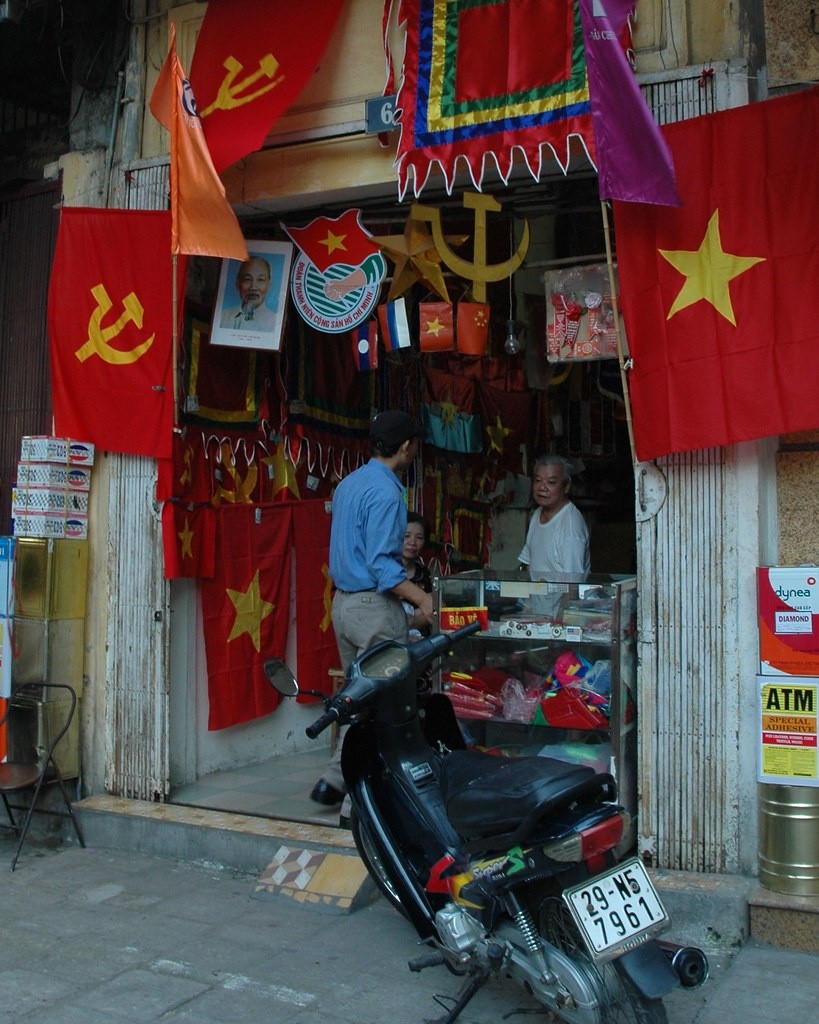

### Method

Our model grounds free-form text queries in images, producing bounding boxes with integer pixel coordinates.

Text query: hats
[369,411,429,448]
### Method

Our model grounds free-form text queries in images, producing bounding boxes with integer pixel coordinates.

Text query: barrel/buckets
[756,783,819,896]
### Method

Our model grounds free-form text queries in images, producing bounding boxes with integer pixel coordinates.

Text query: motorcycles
[261,595,709,1024]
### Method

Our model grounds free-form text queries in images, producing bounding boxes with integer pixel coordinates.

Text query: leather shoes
[311,778,345,806]
[339,815,351,829]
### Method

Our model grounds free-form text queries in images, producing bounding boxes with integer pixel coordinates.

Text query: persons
[516,454,593,667]
[395,510,434,688]
[311,408,447,827]
[221,253,275,334]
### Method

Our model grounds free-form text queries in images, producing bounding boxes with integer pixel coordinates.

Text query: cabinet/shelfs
[433,570,638,860]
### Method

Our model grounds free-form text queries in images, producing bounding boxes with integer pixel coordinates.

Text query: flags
[190,0,346,177]
[148,16,252,261]
[607,86,819,462]
[578,0,685,208]
[161,501,217,580]
[196,500,295,730]
[157,429,214,505]
[291,498,345,705]
[48,204,188,459]
[192,297,624,566]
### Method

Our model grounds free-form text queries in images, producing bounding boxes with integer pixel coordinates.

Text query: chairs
[0,680,87,873]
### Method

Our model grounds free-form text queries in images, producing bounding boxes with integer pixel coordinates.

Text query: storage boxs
[11,435,96,539]
